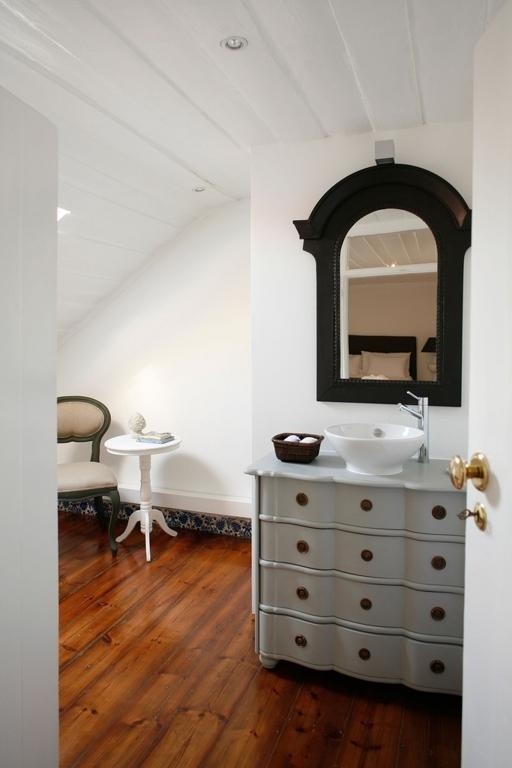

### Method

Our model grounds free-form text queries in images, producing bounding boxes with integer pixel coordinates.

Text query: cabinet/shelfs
[243,469,466,696]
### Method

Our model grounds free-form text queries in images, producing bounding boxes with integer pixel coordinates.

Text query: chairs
[56,396,120,553]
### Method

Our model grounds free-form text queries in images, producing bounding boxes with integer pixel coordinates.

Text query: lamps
[422,337,437,381]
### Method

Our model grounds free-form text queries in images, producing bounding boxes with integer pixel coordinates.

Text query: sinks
[325,420,424,475]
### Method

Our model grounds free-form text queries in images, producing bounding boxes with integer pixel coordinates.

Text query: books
[136,431,175,444]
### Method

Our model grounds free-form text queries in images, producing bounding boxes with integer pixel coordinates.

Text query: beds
[348,335,417,380]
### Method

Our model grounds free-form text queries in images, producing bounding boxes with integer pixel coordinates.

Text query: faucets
[397,390,429,464]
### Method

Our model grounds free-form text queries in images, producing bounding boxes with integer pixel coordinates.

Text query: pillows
[361,351,412,380]
[349,355,361,379]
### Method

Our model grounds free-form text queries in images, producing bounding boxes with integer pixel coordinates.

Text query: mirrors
[293,163,472,407]
